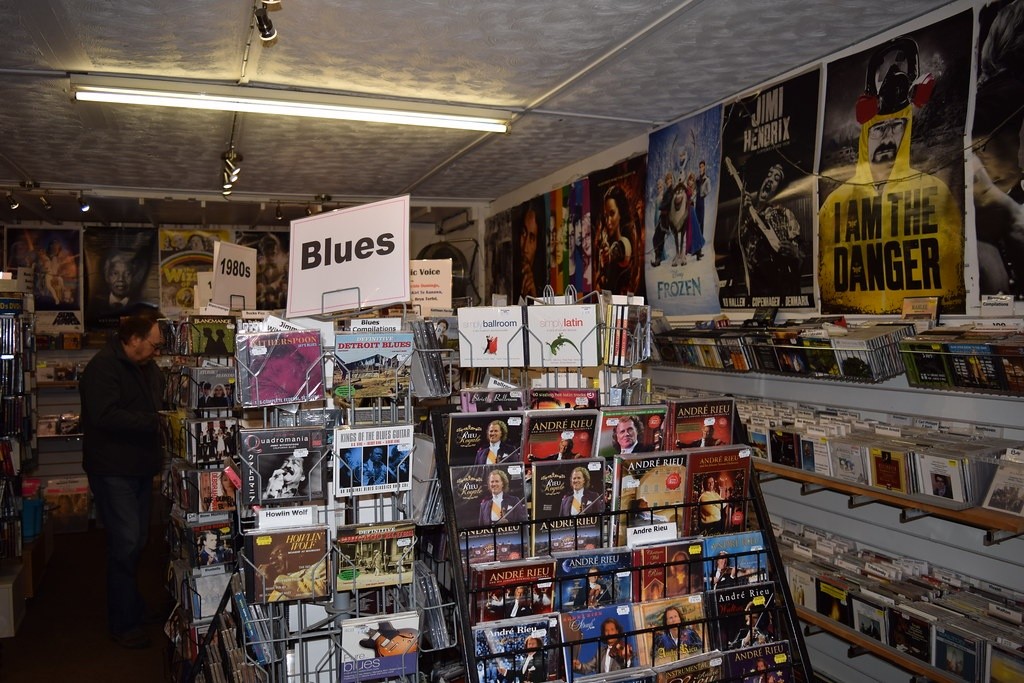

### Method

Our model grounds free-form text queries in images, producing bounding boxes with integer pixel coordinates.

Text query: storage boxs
[41,478,90,534]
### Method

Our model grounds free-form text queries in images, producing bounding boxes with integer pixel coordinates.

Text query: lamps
[78,190,90,212]
[276,200,283,220]
[68,73,512,135]
[221,146,241,195]
[39,190,53,211]
[254,4,278,41]
[5,188,19,210]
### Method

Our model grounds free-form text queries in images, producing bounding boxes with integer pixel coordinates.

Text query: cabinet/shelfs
[0,291,46,638]
[157,317,1024,683]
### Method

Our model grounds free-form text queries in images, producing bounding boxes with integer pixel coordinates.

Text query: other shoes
[108,628,151,649]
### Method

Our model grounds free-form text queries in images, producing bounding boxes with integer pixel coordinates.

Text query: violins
[723,565,747,573]
[587,584,600,608]
[608,640,637,659]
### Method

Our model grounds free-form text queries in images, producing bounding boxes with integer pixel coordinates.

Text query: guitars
[724,155,795,256]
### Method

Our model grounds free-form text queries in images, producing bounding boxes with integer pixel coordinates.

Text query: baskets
[652,341,904,384]
[899,350,1024,398]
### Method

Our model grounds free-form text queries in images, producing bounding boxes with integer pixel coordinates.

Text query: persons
[78,312,175,652]
[188,319,453,597]
[594,183,640,296]
[10,225,289,312]
[513,202,543,307]
[459,415,780,683]
[727,161,806,296]
[818,82,963,313]
[543,197,592,300]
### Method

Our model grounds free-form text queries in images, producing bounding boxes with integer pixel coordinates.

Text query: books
[162,294,1024,683]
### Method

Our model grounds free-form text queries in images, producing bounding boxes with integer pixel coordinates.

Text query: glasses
[868,120,905,139]
[144,337,160,351]
